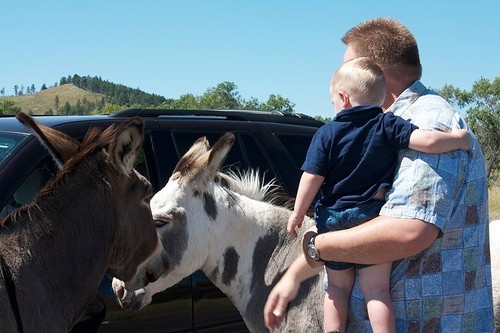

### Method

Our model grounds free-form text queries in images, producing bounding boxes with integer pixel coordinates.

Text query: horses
[1,108,158,333]
[110,132,499,333]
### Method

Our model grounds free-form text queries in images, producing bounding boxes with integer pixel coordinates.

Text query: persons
[287,58,473,333]
[262,19,496,333]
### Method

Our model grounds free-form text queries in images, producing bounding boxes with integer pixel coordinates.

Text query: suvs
[0,110,327,333]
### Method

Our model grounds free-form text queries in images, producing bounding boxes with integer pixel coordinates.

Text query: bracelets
[308,233,324,262]
